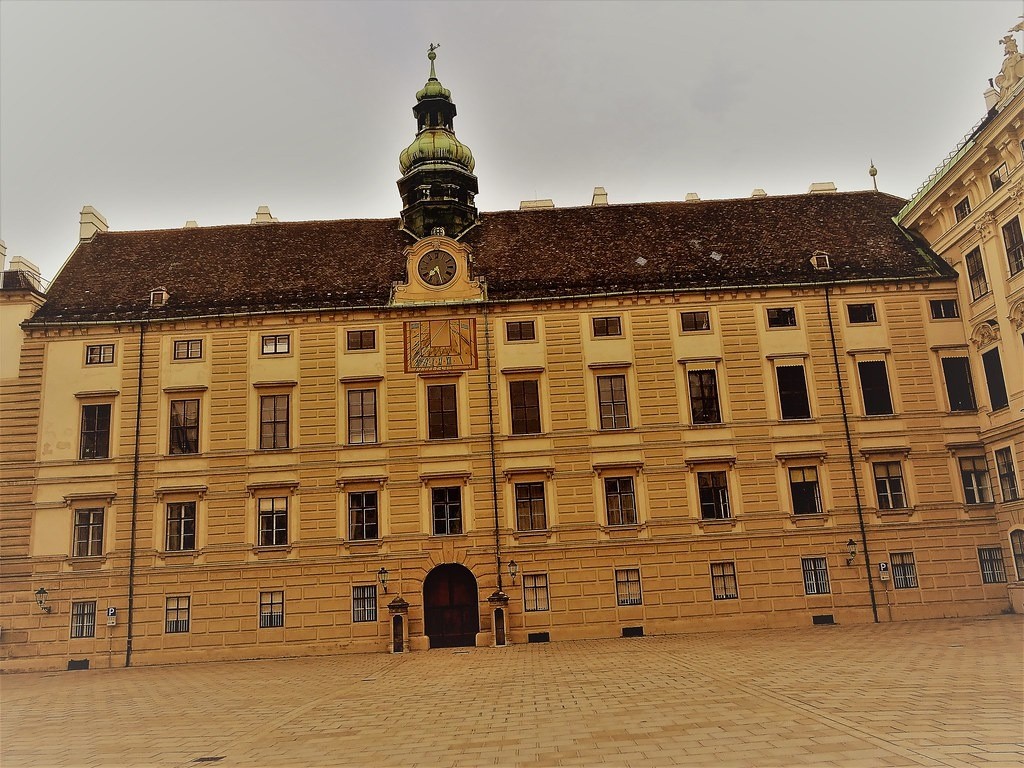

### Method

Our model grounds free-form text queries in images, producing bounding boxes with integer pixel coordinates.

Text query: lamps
[419,250,456,285]
[378,567,388,594]
[846,538,858,566]
[508,560,518,586]
[34,588,51,615]
